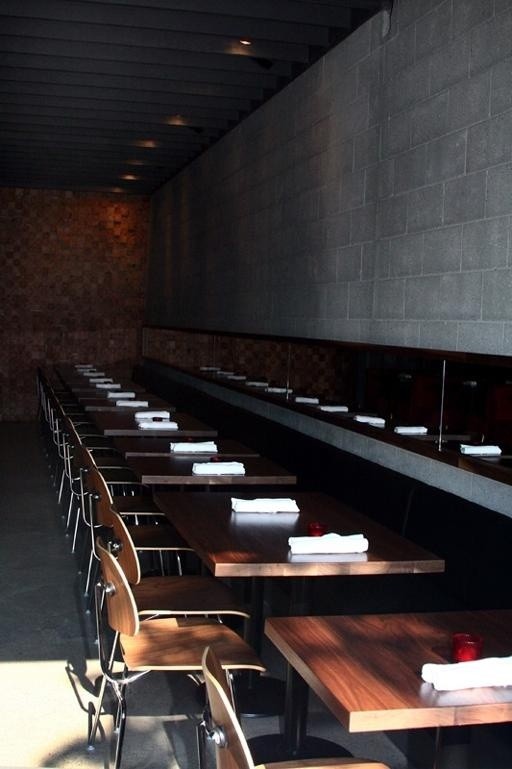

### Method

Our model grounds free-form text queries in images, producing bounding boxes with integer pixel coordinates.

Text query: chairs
[82,532,267,768]
[33,351,512,698]
[195,638,391,769]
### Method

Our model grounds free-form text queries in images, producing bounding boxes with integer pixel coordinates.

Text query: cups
[89,370,195,443]
[450,631,484,661]
[210,456,222,462]
[307,522,328,536]
